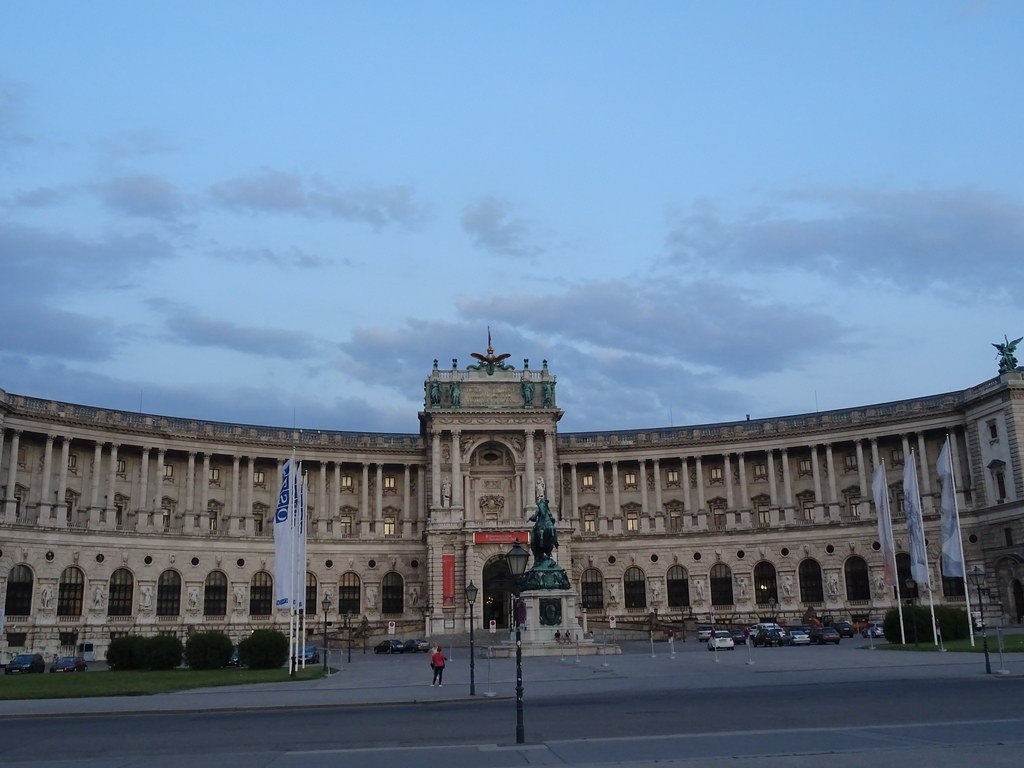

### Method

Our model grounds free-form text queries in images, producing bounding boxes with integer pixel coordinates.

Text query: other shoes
[439,685,442,687]
[430,685,434,687]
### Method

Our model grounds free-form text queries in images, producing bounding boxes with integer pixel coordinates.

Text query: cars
[226,654,244,668]
[728,628,746,646]
[861,621,884,638]
[829,622,855,638]
[404,638,430,654]
[808,627,841,646]
[786,630,811,647]
[292,644,319,667]
[4,652,45,675]
[373,639,406,655]
[750,622,816,640]
[49,656,89,673]
[707,630,735,651]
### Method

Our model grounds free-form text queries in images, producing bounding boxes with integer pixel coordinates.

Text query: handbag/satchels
[430,660,434,670]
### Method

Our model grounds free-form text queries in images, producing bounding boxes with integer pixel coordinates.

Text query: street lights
[362,615,367,655]
[345,609,354,662]
[321,593,332,649]
[904,576,920,648]
[767,596,777,637]
[680,606,686,644]
[502,536,532,745]
[463,578,479,697]
[968,565,992,674]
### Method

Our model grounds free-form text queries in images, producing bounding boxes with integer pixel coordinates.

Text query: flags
[274,455,306,610]
[872,442,962,582]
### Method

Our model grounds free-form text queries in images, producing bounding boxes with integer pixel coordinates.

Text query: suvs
[697,624,715,642]
[753,628,786,648]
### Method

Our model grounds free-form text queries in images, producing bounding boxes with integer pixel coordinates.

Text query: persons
[141,588,152,606]
[738,578,747,597]
[428,642,445,688]
[783,577,792,595]
[555,630,570,645]
[530,499,555,525]
[827,574,838,594]
[536,476,544,497]
[94,587,104,607]
[325,587,332,598]
[190,588,198,606]
[522,379,555,404]
[369,588,375,606]
[450,378,462,406]
[235,588,244,608]
[410,588,418,604]
[873,571,884,591]
[431,378,440,404]
[696,581,703,598]
[442,476,451,496]
[652,582,660,600]
[609,584,617,601]
[43,585,55,609]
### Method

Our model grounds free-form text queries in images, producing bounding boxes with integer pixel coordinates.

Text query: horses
[530,500,557,557]
